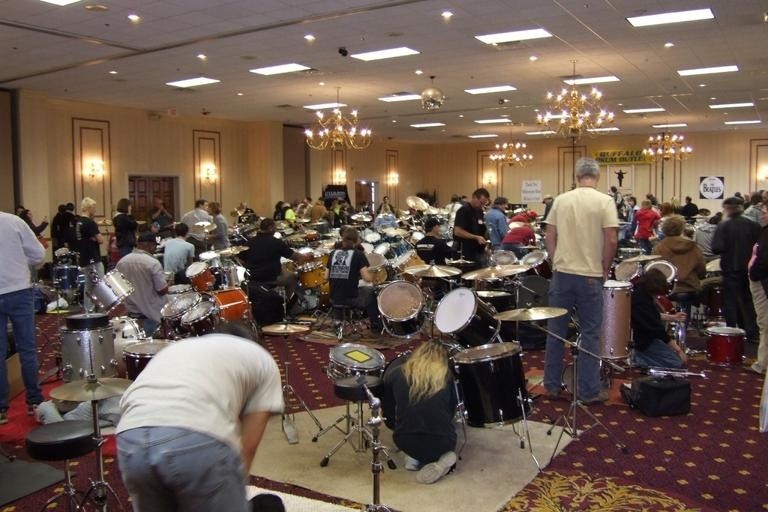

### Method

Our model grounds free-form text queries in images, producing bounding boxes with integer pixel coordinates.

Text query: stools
[317,305,370,342]
[244,282,287,325]
[313,375,397,470]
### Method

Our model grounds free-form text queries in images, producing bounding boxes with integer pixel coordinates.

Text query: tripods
[74,402,127,511]
[547,345,629,467]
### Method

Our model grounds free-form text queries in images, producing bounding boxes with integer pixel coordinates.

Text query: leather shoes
[544,387,571,399]
[585,392,609,403]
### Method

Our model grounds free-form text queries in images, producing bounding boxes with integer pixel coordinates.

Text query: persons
[627,271,686,371]
[245,219,313,321]
[52,202,67,253]
[311,197,327,223]
[19,209,49,286]
[324,226,383,335]
[539,189,551,233]
[113,321,284,512]
[332,195,343,214]
[146,196,174,239]
[744,194,765,233]
[279,202,303,224]
[324,201,340,227]
[709,194,760,344]
[377,196,395,214]
[335,224,364,253]
[756,188,767,220]
[652,216,706,306]
[414,219,461,266]
[296,197,313,217]
[207,203,234,252]
[625,194,639,241]
[487,197,510,248]
[742,191,750,206]
[75,195,106,315]
[459,195,467,205]
[609,184,626,223]
[271,203,285,222]
[646,192,659,218]
[356,200,372,216]
[182,199,216,258]
[531,157,619,406]
[743,200,768,375]
[61,200,78,254]
[0,202,58,425]
[381,338,461,485]
[444,193,464,229]
[694,215,719,258]
[372,204,399,230]
[230,200,258,224]
[13,206,25,216]
[339,198,354,227]
[680,195,700,223]
[500,224,534,262]
[112,199,135,256]
[659,199,675,240]
[149,222,162,236]
[449,188,494,266]
[118,233,171,332]
[410,210,423,227]
[633,200,659,255]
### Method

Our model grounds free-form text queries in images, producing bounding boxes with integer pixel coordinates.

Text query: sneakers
[405,456,421,471]
[417,451,457,484]
[0,413,8,424]
[27,405,34,416]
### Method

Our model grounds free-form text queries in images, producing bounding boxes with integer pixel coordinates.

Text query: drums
[363,231,382,245]
[159,290,204,343]
[440,206,452,222]
[409,231,424,244]
[167,283,193,303]
[179,300,218,338]
[53,265,81,291]
[381,227,409,240]
[449,340,536,428]
[326,341,388,390]
[84,267,138,316]
[185,261,218,291]
[222,264,241,286]
[503,275,550,349]
[614,259,643,284]
[652,293,679,334]
[199,250,221,268]
[210,287,253,324]
[392,249,426,279]
[645,258,677,286]
[293,247,329,267]
[164,271,176,286]
[279,259,295,273]
[490,250,517,265]
[319,282,331,310]
[377,241,397,261]
[298,261,331,288]
[110,314,147,377]
[56,324,118,383]
[305,227,320,241]
[55,247,71,265]
[599,279,633,360]
[704,325,748,366]
[357,241,375,253]
[434,286,502,348]
[376,278,431,340]
[520,250,554,279]
[318,238,336,250]
[363,252,396,288]
[709,285,726,321]
[123,338,176,381]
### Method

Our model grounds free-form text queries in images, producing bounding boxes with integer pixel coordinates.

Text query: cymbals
[213,244,249,257]
[351,214,373,222]
[520,243,540,252]
[402,263,463,278]
[48,374,134,402]
[230,204,248,216]
[475,288,514,298]
[446,255,475,265]
[95,219,117,228]
[616,246,645,254]
[312,218,330,226]
[292,216,310,222]
[704,257,722,271]
[458,264,531,281]
[422,204,439,215]
[624,253,663,262]
[260,321,312,335]
[689,214,709,219]
[405,195,429,211]
[491,305,570,322]
[134,218,151,226]
[193,220,218,233]
[697,207,710,212]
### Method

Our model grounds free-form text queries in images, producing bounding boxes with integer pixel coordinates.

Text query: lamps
[533,85,618,142]
[488,137,535,168]
[420,88,445,111]
[642,131,693,162]
[303,106,372,152]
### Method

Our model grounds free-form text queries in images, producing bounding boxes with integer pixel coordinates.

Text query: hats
[137,231,160,245]
[723,197,745,206]
[425,218,444,230]
[542,194,554,203]
